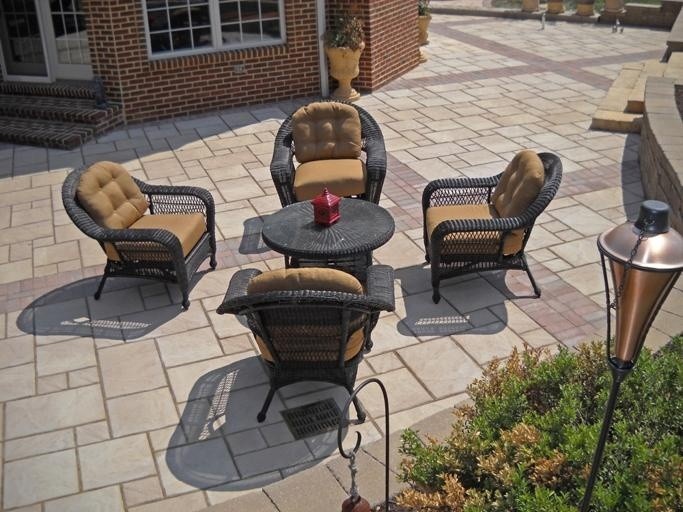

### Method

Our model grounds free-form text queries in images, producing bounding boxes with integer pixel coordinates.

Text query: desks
[262,198,395,281]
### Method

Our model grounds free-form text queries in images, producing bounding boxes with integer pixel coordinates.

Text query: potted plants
[319,10,366,103]
[418,0,432,47]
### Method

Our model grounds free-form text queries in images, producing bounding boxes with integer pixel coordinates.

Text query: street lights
[580,199,683,512]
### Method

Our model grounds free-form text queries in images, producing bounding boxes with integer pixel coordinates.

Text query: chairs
[269,100,387,208]
[62,160,217,310]
[422,152,563,303]
[215,264,394,422]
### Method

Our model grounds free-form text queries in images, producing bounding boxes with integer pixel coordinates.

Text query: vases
[521,0,624,16]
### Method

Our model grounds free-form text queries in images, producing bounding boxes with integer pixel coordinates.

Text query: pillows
[77,160,151,228]
[291,103,362,163]
[246,266,364,335]
[491,150,546,236]
